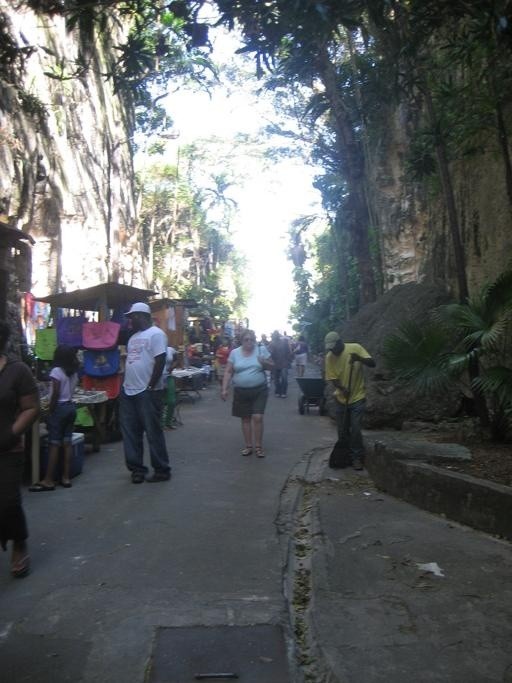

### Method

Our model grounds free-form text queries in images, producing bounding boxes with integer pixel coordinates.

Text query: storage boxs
[40,433,85,483]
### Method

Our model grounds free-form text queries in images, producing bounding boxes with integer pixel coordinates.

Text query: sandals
[241,447,252,456]
[255,446,265,457]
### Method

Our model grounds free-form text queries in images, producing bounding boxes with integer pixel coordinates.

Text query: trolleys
[296,377,326,415]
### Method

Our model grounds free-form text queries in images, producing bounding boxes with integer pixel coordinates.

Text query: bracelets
[146,385,155,391]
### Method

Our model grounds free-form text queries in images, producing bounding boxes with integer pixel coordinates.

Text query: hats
[324,332,339,349]
[124,303,150,314]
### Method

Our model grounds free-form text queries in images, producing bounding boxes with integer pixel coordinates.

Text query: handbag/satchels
[36,317,120,400]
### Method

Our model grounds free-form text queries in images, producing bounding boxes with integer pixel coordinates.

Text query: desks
[29,390,108,492]
[172,368,202,404]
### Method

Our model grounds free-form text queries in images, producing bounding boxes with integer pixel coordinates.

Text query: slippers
[62,480,72,488]
[29,483,55,491]
[11,548,30,578]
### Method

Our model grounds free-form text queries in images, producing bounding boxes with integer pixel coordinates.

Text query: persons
[27,343,81,492]
[220,330,277,458]
[322,330,377,470]
[188,334,311,399]
[118,301,172,483]
[0,317,41,577]
[158,346,182,431]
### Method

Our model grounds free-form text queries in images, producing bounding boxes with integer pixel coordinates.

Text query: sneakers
[133,472,144,483]
[148,473,170,482]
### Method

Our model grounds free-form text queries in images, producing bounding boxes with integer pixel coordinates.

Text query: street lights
[105,127,180,281]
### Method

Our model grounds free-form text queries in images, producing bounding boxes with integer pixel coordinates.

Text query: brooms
[328,362,355,470]
